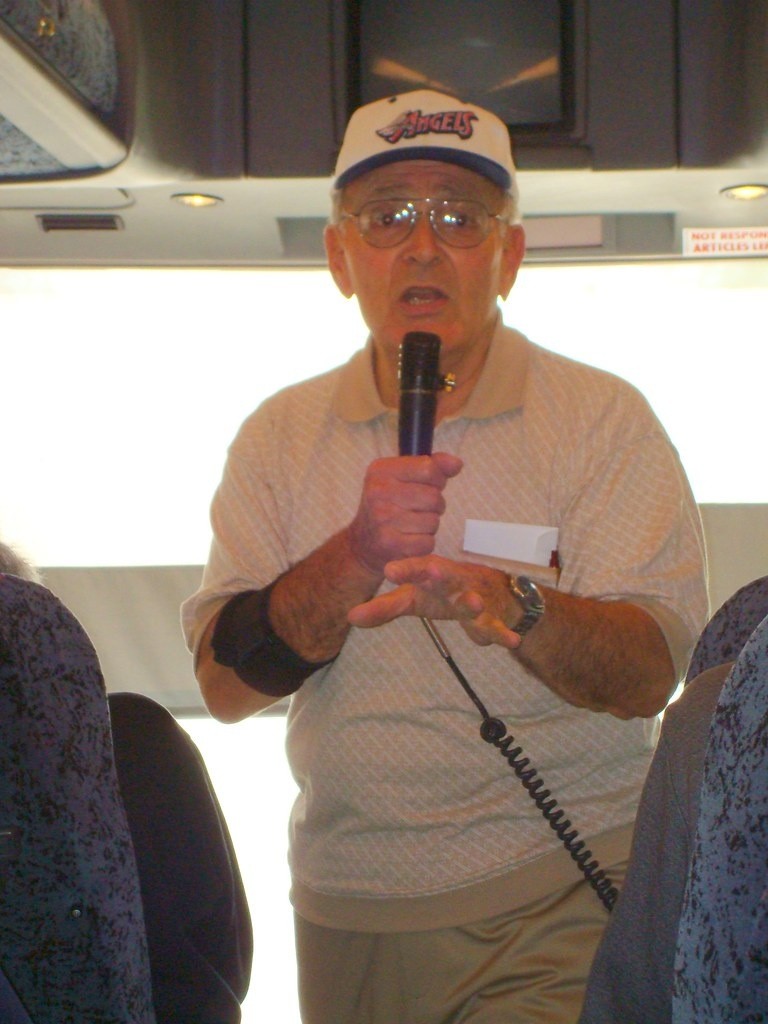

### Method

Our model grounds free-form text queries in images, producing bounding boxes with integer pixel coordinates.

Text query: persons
[181,89,710,1024]
[578,662,735,1024]
[0,541,253,1024]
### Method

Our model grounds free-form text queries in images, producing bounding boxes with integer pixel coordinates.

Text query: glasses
[342,197,512,249]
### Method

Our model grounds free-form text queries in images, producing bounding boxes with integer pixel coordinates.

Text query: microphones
[398,331,441,456]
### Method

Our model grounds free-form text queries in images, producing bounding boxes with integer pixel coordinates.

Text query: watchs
[505,571,547,634]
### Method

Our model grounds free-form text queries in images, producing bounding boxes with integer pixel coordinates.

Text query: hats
[334,90,519,216]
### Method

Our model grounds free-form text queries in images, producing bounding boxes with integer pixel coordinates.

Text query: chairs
[683,574,768,688]
[107,692,255,1024]
[0,577,156,1024]
[665,610,768,1024]
